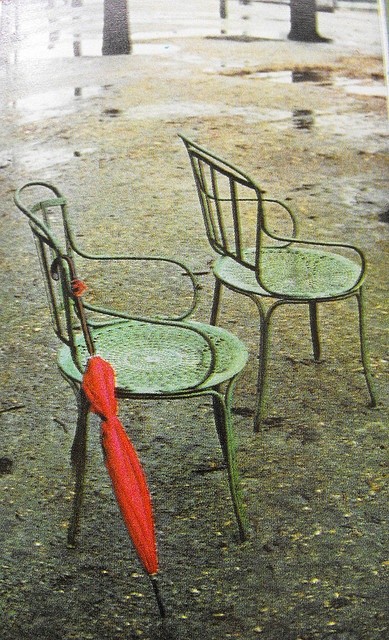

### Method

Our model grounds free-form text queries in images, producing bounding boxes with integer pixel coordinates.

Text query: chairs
[15,180,253,548]
[177,133,383,431]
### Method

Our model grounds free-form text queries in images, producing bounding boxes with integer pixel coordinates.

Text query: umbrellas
[50,255,165,620]
